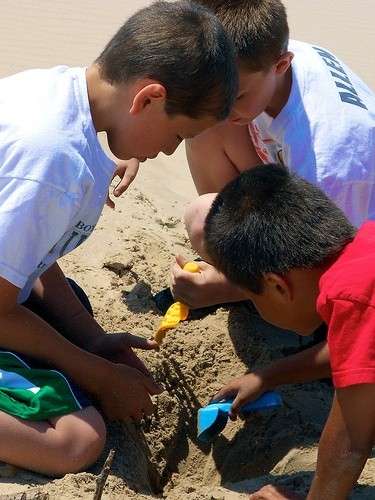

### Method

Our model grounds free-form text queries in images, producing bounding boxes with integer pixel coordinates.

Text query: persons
[151,1,375,320]
[203,163,375,500]
[0,0,239,478]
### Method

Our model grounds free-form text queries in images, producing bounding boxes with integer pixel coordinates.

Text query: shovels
[198,390,285,443]
[154,262,202,346]
